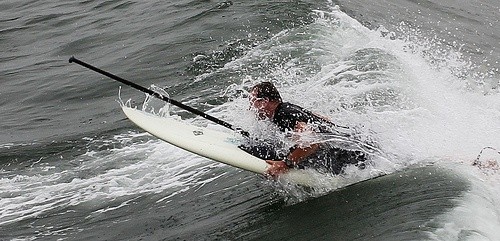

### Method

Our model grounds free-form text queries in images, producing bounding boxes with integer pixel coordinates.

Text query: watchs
[283,157,293,167]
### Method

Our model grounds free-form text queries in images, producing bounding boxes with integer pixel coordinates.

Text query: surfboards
[121,104,339,190]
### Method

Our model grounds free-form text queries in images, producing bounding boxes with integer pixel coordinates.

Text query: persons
[247,83,376,177]
